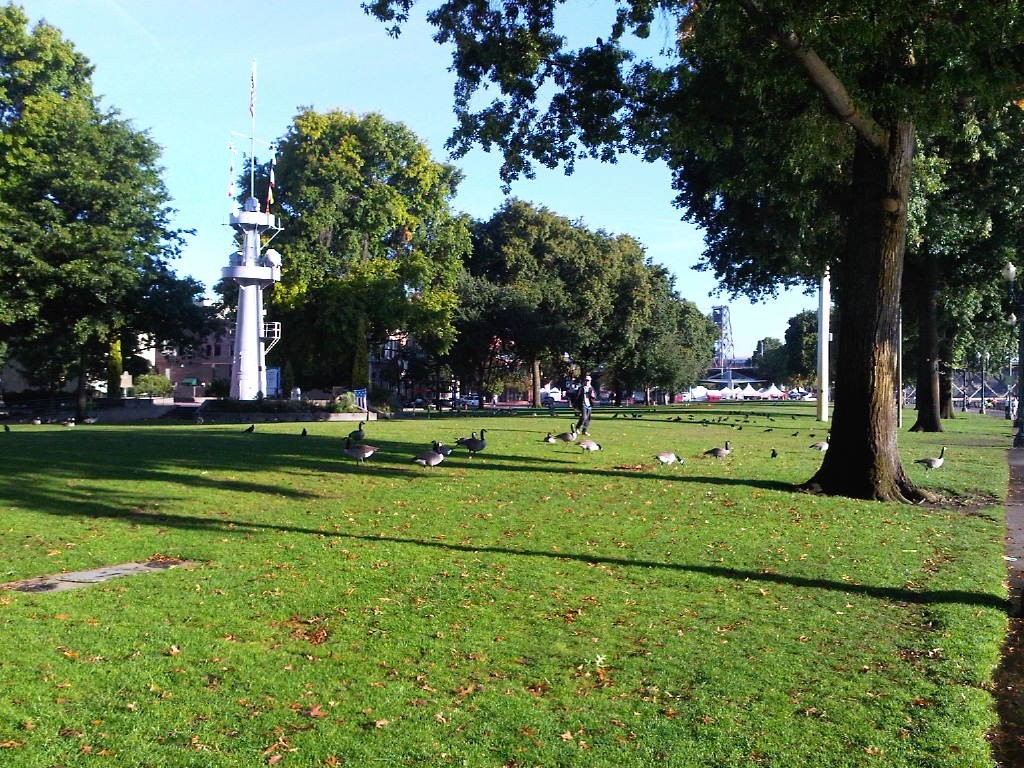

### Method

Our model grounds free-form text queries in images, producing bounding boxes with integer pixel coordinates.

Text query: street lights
[976,352,990,414]
[1006,313,1017,420]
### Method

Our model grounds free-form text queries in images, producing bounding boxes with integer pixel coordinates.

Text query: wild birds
[464,401,832,465]
[341,421,380,468]
[914,446,949,472]
[244,425,254,433]
[410,440,452,471]
[449,429,487,456]
[302,428,306,436]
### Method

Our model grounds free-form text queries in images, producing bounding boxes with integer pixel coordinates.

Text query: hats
[584,375,592,381]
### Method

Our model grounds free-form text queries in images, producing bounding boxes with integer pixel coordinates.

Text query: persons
[987,395,1019,416]
[574,375,596,436]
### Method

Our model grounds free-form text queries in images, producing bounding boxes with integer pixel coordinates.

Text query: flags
[228,139,236,195]
[266,150,277,206]
[249,62,257,116]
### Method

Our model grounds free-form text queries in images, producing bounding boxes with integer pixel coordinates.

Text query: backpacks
[566,386,584,408]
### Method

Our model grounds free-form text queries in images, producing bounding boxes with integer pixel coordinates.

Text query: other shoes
[575,428,581,435]
[582,431,591,436]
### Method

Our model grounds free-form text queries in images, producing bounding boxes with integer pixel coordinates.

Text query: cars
[789,392,797,398]
[403,398,425,408]
[462,396,471,406]
[460,395,467,400]
[466,396,479,405]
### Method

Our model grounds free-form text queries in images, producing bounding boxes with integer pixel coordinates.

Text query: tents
[720,382,805,400]
